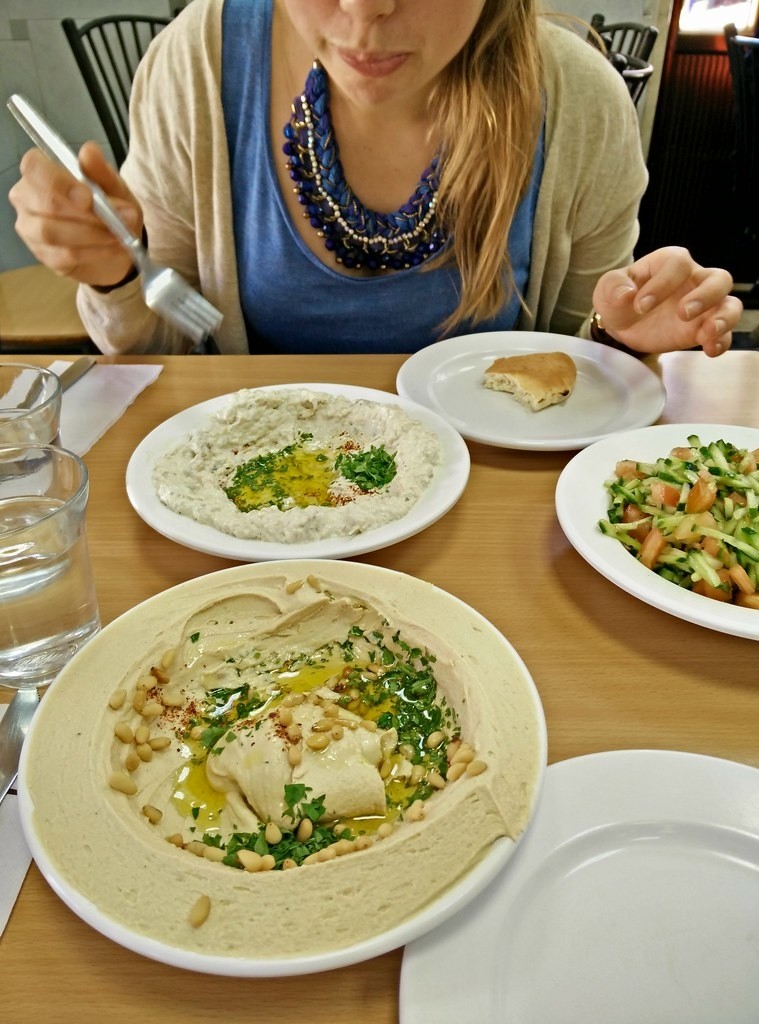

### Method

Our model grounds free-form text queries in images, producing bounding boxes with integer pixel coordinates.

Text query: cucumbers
[598,436,759,591]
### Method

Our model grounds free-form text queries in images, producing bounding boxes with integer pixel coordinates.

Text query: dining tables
[0,349,758,1024]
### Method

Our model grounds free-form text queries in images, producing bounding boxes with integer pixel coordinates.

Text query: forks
[7,94,224,341]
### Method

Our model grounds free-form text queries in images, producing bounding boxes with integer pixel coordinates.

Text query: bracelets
[590,312,602,344]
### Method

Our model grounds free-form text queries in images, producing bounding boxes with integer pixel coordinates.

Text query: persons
[7,0,743,358]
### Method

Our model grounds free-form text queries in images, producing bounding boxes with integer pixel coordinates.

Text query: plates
[17,558,549,980]
[398,747,759,1023]
[396,330,669,452]
[123,382,473,563]
[555,422,759,641]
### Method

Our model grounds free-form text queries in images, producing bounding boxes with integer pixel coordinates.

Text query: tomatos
[612,447,759,610]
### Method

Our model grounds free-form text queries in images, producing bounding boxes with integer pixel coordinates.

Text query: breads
[484,351,579,409]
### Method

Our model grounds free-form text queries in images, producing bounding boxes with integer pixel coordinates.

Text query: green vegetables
[172,623,464,870]
[220,431,398,512]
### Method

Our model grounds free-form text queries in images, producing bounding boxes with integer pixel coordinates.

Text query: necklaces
[283,60,454,275]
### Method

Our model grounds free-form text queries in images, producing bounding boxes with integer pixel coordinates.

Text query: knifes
[1,684,41,805]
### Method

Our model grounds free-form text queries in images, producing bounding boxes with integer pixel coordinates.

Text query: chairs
[724,26,759,292]
[585,11,659,101]
[58,14,179,168]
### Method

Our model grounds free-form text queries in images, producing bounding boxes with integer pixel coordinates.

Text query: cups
[1,363,61,443]
[0,444,103,688]
